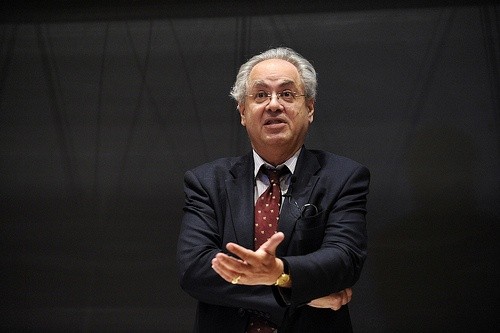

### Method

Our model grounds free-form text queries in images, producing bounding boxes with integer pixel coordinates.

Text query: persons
[176,48,372,333]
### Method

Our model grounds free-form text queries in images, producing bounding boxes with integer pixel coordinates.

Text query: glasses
[242,89,310,102]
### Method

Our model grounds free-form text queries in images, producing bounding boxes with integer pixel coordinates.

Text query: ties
[248,164,291,332]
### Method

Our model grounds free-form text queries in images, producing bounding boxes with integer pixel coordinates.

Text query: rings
[231,276,242,285]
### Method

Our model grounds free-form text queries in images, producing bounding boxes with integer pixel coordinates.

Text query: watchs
[275,258,290,287]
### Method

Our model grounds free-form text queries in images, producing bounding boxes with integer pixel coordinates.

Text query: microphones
[287,175,297,196]
